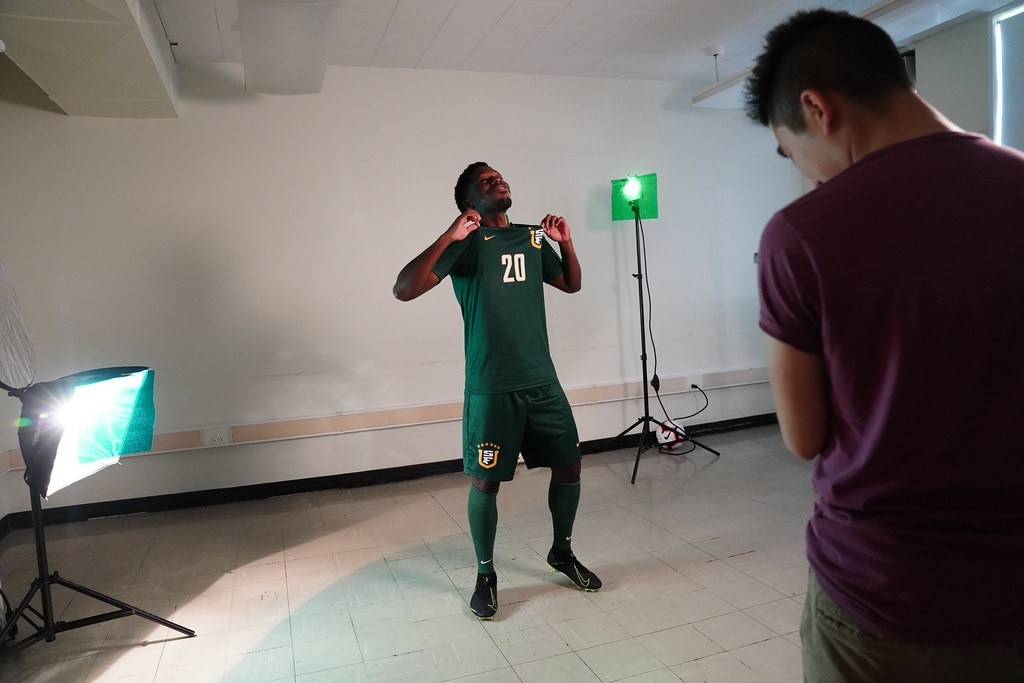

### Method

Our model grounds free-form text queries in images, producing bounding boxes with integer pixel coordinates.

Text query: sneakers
[546,546,603,592]
[469,570,499,620]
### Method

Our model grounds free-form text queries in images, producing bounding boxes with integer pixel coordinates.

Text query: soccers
[657,420,686,450]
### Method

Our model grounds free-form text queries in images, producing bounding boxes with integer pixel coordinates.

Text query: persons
[392,160,604,619]
[740,5,1024,683]
